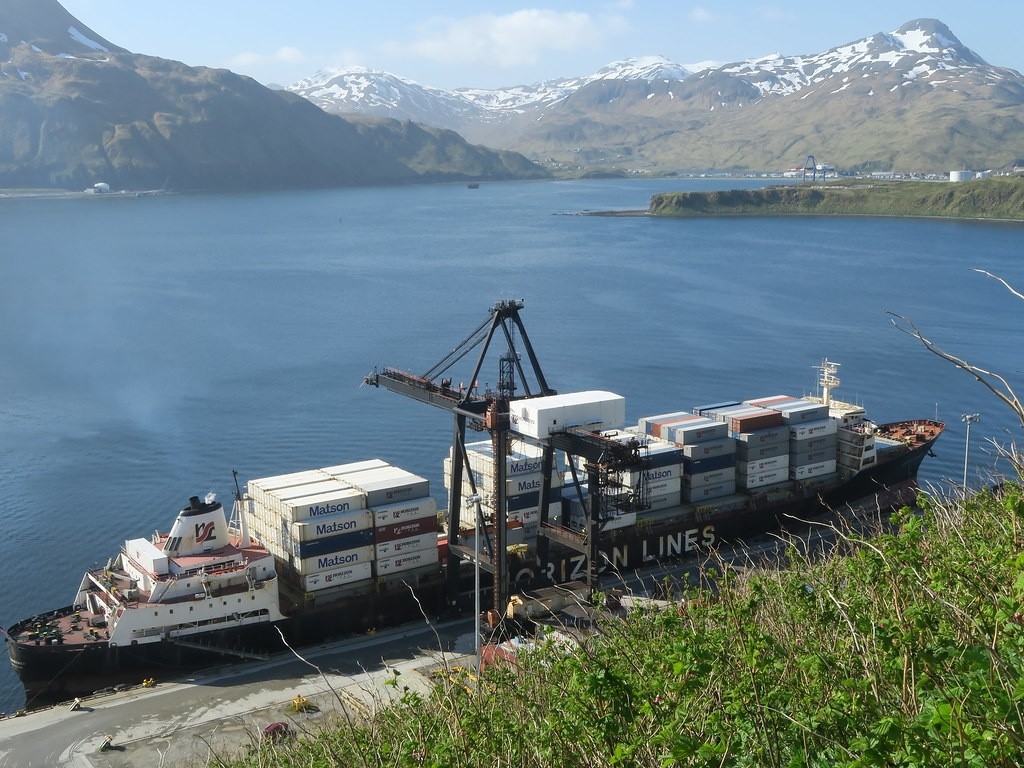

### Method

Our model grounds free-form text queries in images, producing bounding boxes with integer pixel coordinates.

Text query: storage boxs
[237,390,877,676]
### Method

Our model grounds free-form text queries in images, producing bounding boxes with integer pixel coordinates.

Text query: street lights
[463,492,498,652]
[961,412,981,499]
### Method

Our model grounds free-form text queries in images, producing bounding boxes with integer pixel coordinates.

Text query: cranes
[357,299,650,640]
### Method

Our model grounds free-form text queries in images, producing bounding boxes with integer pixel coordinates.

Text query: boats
[6,351,946,709]
